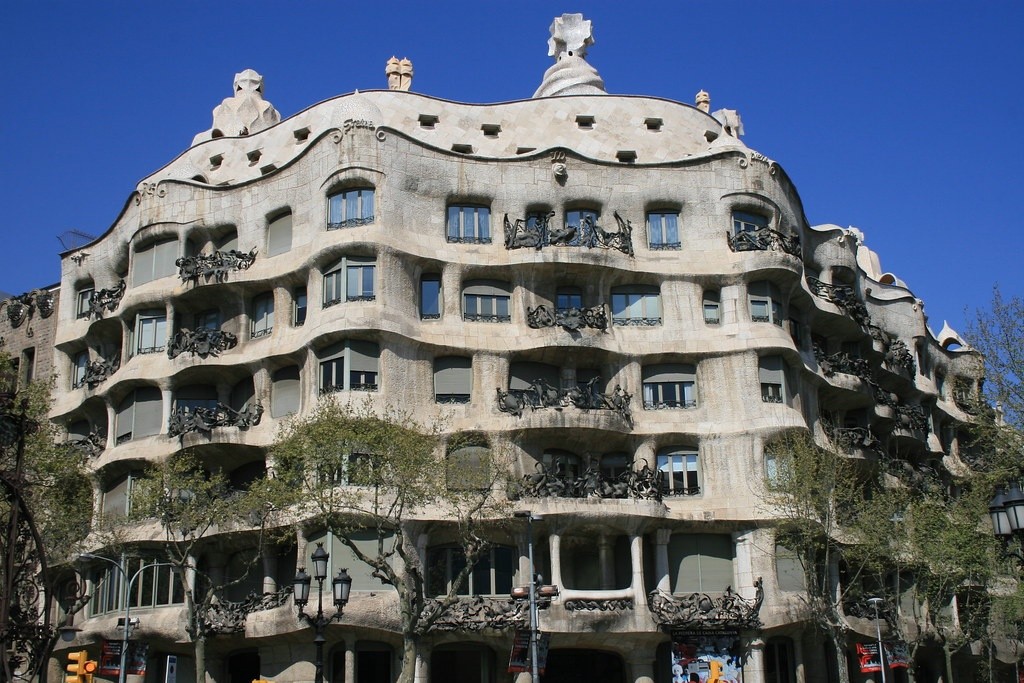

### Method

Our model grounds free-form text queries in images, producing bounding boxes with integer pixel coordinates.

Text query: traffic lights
[83,660,97,674]
[66,651,88,683]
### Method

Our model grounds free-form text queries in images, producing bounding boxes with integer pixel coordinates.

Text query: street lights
[868,598,888,683]
[79,552,187,683]
[291,542,353,683]
[510,509,559,683]
